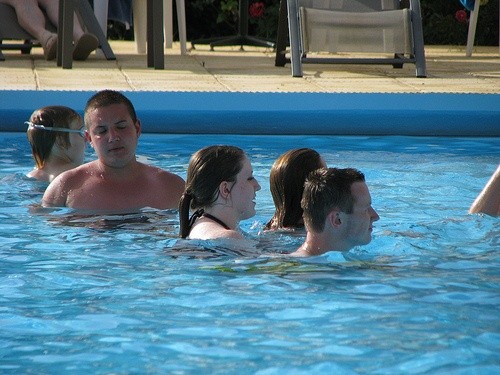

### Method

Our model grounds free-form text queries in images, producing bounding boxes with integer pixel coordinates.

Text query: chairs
[0,0,480,78]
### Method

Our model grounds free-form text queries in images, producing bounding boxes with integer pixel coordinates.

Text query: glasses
[29,121,86,137]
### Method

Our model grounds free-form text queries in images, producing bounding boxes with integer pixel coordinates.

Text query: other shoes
[73,32,99,61]
[44,33,60,61]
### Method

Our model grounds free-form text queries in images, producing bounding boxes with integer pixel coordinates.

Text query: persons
[260,148,327,234]
[26,106,87,182]
[177,146,262,242]
[41,90,187,210]
[289,167,380,259]
[469,164,500,217]
[3,0,99,60]
